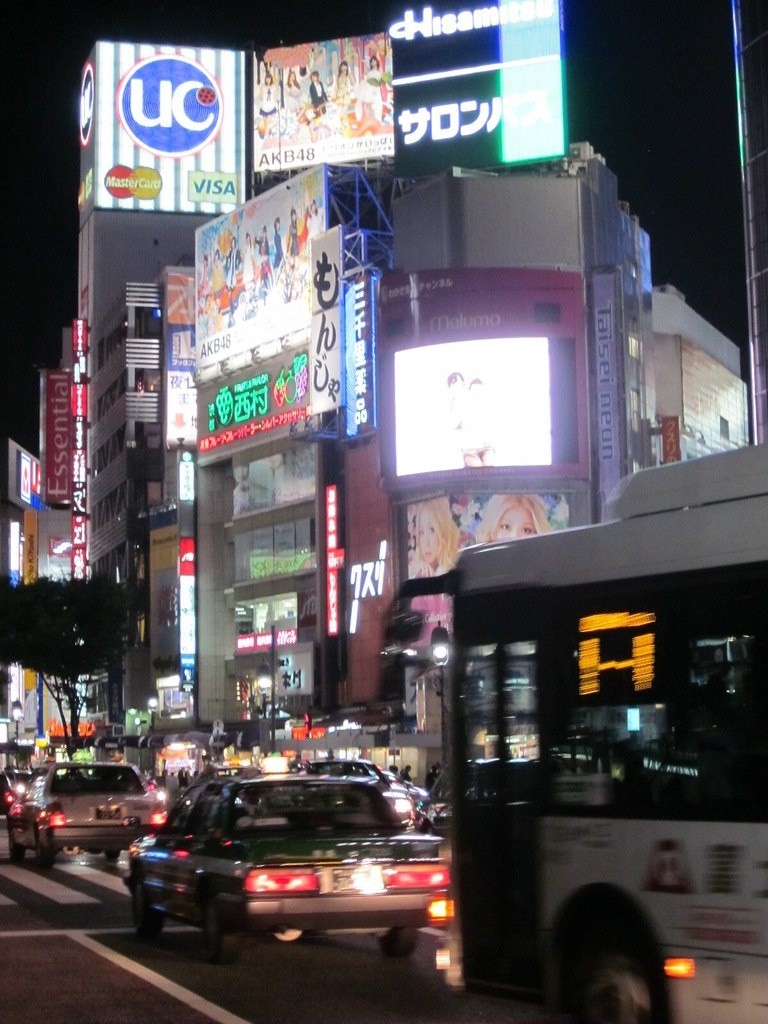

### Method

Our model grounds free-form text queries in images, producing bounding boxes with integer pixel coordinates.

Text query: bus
[383,443,768,1023]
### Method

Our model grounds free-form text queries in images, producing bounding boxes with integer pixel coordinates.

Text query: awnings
[68,732,244,751]
[0,743,19,753]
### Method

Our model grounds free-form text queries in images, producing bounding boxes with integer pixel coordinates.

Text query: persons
[199,253,213,319]
[437,369,508,469]
[390,763,442,791]
[475,493,551,545]
[411,497,461,647]
[309,69,336,138]
[285,201,323,300]
[331,60,355,136]
[224,238,242,328]
[241,233,257,311]
[211,249,225,332]
[356,56,382,117]
[176,768,199,793]
[270,217,286,281]
[284,72,316,143]
[256,226,273,307]
[233,465,253,516]
[258,73,277,139]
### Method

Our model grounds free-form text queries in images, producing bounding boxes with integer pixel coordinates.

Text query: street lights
[258,659,272,757]
[430,620,450,764]
[13,701,22,770]
[147,688,159,779]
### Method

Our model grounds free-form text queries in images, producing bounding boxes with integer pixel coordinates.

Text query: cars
[124,775,454,966]
[297,759,431,832]
[7,762,167,866]
[410,758,531,869]
[0,770,32,815]
